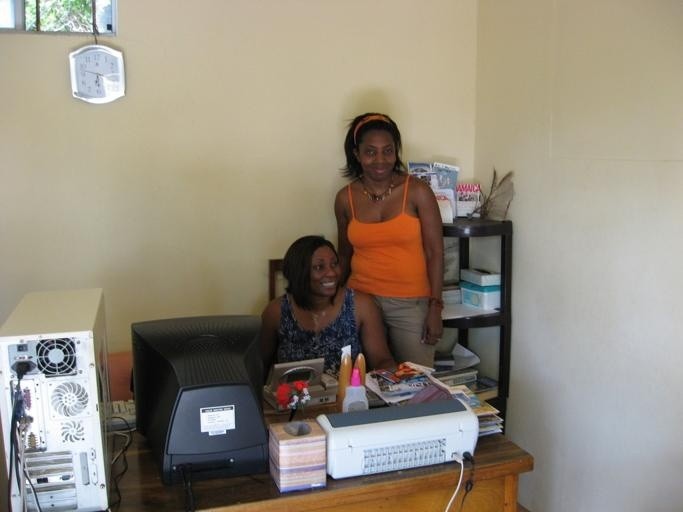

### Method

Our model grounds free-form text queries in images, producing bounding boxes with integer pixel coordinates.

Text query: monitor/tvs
[130,316,270,486]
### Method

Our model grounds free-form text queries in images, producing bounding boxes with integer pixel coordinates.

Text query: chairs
[267,257,289,300]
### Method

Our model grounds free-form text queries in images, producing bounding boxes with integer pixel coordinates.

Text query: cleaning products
[342,367,369,414]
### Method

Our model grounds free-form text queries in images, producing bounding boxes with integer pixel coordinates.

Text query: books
[365,360,504,437]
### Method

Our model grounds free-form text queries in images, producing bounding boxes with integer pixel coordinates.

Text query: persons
[334,112,444,367]
[258,235,396,385]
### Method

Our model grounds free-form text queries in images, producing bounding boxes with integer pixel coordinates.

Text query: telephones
[263,357,338,411]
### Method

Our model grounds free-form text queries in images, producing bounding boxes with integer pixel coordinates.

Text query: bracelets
[429,296,444,309]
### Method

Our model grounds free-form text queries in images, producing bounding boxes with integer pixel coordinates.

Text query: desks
[109,373,534,511]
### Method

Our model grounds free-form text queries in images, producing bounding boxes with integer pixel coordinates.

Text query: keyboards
[105,398,137,425]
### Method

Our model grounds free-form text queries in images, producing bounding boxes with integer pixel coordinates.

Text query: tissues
[268,418,327,493]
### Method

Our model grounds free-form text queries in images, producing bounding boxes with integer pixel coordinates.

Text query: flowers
[275,374,311,414]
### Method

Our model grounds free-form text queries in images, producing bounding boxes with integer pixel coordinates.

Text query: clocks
[67,44,127,106]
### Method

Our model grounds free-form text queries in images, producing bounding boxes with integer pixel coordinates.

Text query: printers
[316,397,479,481]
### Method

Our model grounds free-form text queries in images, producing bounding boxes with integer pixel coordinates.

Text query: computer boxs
[0,286,109,511]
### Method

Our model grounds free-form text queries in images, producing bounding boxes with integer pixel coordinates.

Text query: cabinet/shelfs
[435,215,513,434]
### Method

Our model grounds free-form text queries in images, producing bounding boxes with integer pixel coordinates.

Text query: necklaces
[359,177,395,201]
